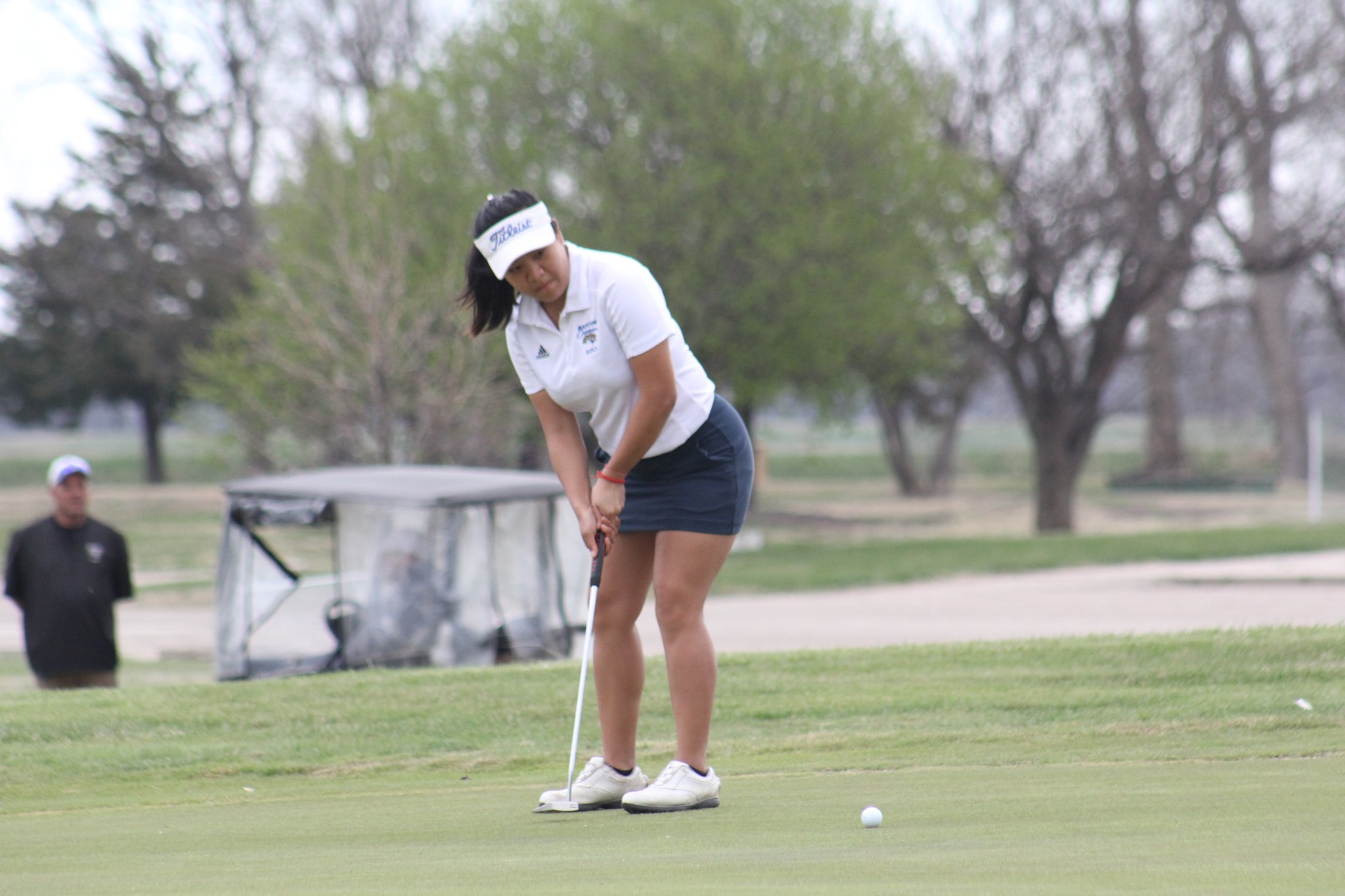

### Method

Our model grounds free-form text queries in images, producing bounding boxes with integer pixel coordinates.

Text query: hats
[473,200,556,280]
[47,454,90,487]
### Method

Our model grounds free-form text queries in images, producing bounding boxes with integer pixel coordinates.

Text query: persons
[458,189,755,812]
[3,457,135,689]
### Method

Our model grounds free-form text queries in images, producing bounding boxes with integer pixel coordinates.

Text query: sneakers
[539,757,648,812]
[620,761,720,814]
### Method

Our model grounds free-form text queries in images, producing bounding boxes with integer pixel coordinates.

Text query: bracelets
[592,471,625,484]
[604,464,627,477]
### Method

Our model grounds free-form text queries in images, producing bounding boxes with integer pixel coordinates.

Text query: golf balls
[861,806,883,828]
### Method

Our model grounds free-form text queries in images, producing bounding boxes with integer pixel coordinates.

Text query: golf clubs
[531,529,605,813]
